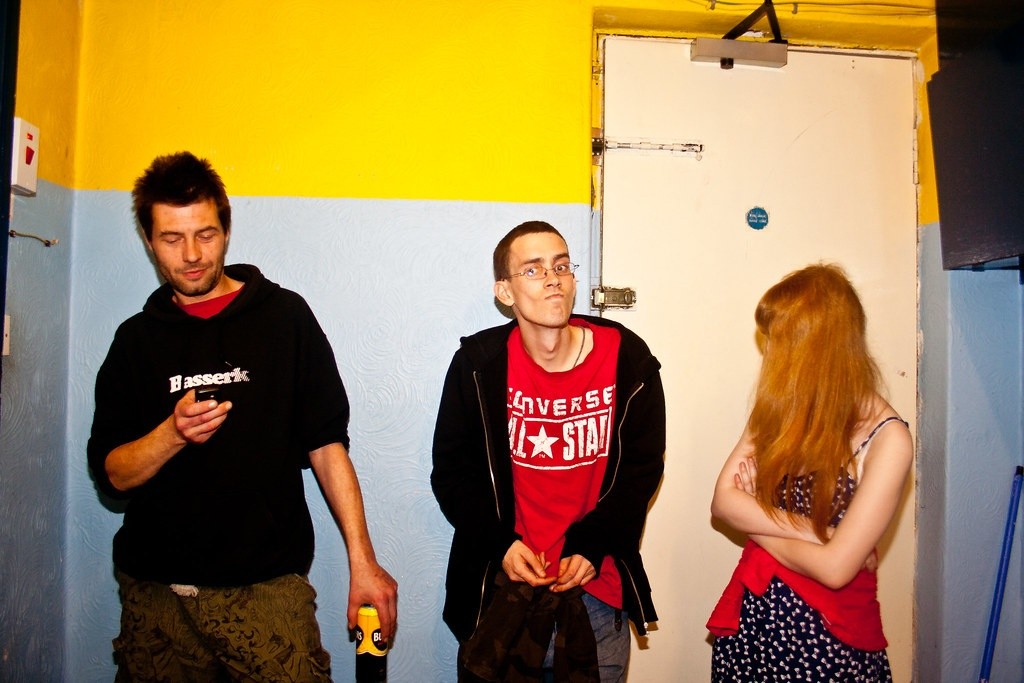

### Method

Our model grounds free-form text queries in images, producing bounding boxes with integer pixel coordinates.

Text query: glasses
[501,263,580,280]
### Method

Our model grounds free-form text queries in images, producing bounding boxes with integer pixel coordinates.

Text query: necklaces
[573,326,586,370]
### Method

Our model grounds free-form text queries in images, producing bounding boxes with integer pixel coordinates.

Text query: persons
[431,221,668,682]
[88,152,397,683]
[706,256,913,683]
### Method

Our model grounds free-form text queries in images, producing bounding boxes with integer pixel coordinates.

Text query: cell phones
[195,387,223,404]
